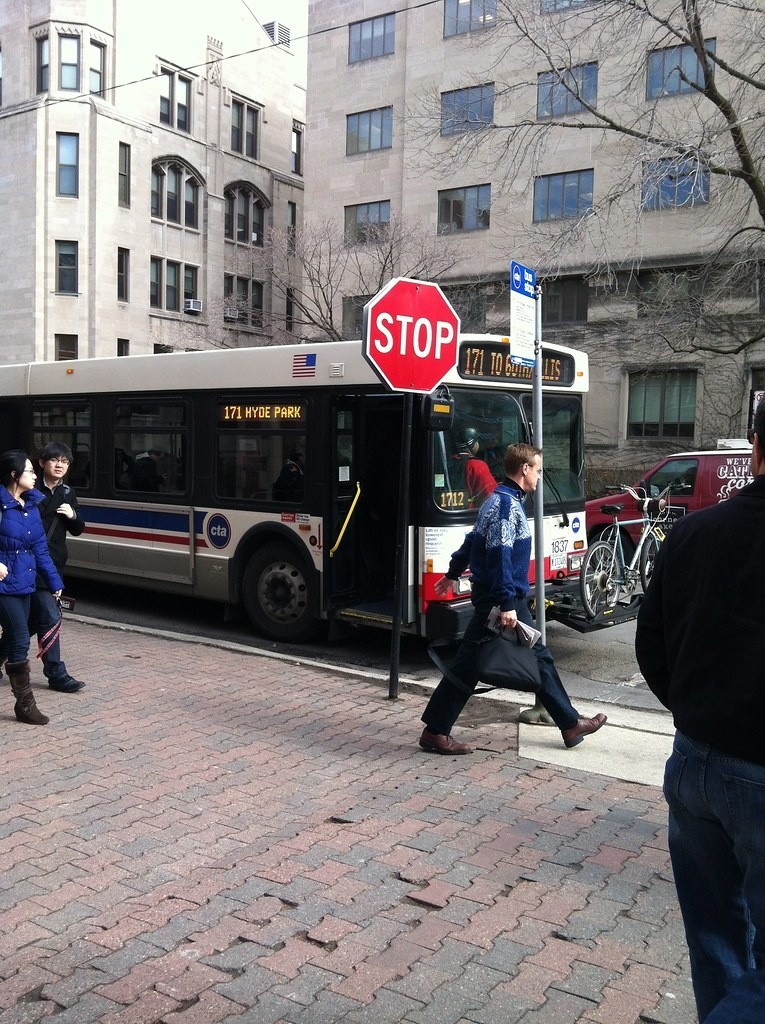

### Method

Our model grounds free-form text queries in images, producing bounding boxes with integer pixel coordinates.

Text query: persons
[419,442,607,755]
[445,419,507,509]
[0,440,87,724]
[634,393,765,1024]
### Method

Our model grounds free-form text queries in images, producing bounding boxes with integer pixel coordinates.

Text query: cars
[586,439,755,575]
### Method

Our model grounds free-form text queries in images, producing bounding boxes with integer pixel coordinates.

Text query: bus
[0,333,591,647]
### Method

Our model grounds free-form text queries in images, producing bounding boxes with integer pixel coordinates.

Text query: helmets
[454,426,480,449]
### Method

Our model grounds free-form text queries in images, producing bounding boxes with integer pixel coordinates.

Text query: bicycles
[579,483,692,620]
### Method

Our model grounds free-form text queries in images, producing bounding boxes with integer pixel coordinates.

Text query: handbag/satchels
[427,625,542,694]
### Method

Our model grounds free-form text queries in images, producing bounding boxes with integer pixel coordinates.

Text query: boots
[5,660,50,725]
[0,638,8,679]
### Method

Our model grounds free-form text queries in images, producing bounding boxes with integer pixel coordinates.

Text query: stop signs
[366,276,460,394]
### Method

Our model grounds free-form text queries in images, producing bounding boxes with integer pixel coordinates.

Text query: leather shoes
[561,713,607,748]
[47,673,86,694]
[419,727,471,755]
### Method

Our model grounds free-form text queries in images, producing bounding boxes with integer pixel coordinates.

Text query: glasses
[746,429,756,444]
[50,457,69,464]
[520,463,542,474]
[23,470,35,474]
